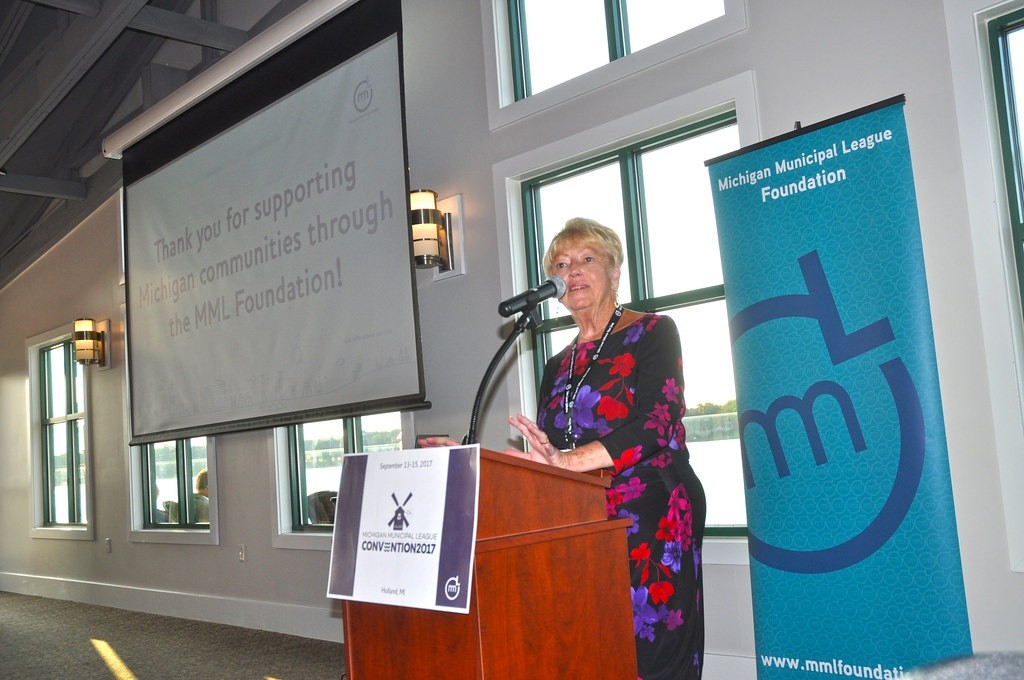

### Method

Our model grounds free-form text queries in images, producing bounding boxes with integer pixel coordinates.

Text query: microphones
[498,274,568,318]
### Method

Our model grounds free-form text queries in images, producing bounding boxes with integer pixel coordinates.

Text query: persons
[418,218,706,680]
[155,484,169,523]
[192,468,210,523]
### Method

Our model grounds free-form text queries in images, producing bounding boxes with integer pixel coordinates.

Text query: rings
[540,435,549,444]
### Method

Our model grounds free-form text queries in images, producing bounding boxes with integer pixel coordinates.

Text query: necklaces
[579,317,609,339]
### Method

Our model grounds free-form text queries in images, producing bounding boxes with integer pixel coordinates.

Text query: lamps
[72,318,110,372]
[410,189,466,283]
